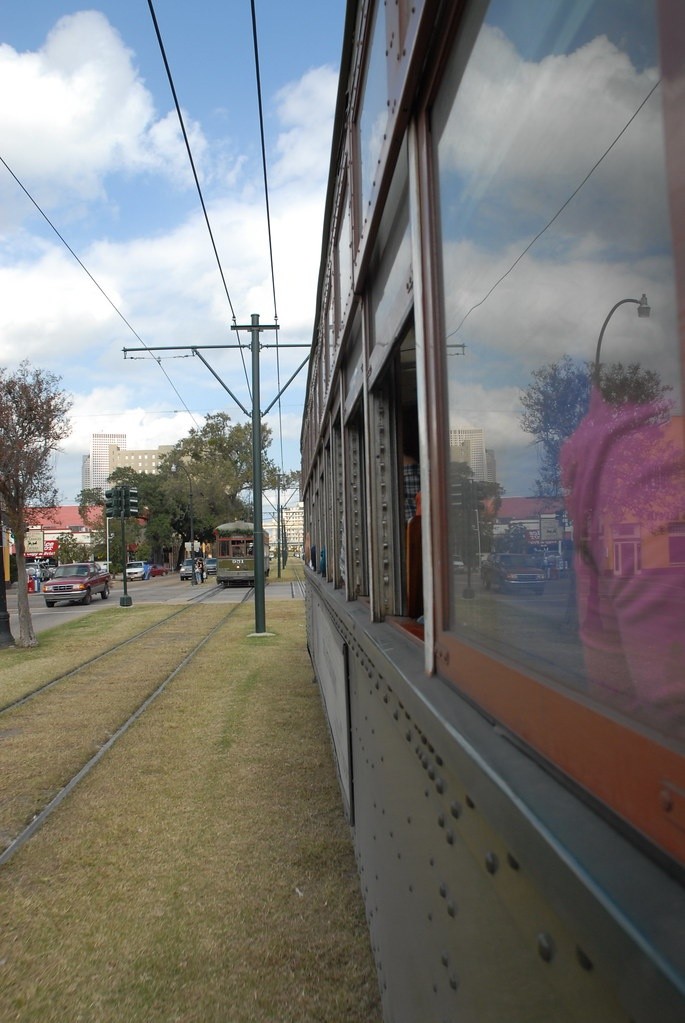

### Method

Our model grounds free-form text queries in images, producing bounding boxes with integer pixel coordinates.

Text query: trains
[298,0,684,1023]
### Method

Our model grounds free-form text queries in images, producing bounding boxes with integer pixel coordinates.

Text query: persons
[143,562,151,580]
[194,557,204,585]
[559,316,685,716]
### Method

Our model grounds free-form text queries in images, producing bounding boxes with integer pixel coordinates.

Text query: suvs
[179,558,209,582]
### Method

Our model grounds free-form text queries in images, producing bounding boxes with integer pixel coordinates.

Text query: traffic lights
[124,486,139,518]
[105,485,119,519]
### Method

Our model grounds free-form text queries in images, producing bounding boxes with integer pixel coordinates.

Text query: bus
[216,522,271,586]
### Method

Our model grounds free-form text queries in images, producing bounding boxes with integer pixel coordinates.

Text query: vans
[126,561,148,580]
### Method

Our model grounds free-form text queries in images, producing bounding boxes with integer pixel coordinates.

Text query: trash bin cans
[34,577,40,593]
[27,575,35,593]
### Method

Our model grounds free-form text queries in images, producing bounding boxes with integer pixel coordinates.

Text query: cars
[480,551,547,596]
[148,564,168,577]
[203,558,218,575]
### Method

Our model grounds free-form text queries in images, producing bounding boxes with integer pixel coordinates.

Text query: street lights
[591,294,651,400]
[170,464,199,586]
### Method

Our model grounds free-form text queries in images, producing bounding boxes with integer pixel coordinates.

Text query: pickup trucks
[43,563,112,608]
